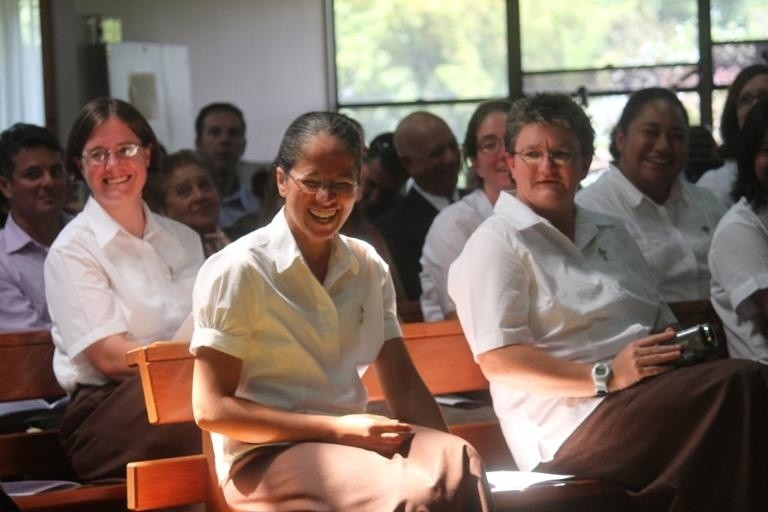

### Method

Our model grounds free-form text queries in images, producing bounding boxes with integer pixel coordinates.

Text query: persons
[44,96,206,485]
[359,132,411,218]
[420,99,513,322]
[339,219,407,303]
[574,86,728,301]
[705,95,768,365]
[683,124,724,181]
[447,89,768,512]
[189,110,493,511]
[0,120,76,332]
[195,102,261,239]
[368,111,467,300]
[152,148,231,255]
[695,64,768,210]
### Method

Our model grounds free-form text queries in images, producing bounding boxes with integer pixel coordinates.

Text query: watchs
[591,363,614,395]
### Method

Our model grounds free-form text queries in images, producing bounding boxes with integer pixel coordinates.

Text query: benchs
[0,333,125,510]
[127,298,722,512]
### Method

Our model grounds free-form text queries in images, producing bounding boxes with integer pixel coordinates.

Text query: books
[1,480,83,495]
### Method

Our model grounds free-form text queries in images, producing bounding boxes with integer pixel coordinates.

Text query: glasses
[510,146,583,163]
[733,91,766,106]
[82,144,141,167]
[476,135,507,154]
[287,170,358,197]
